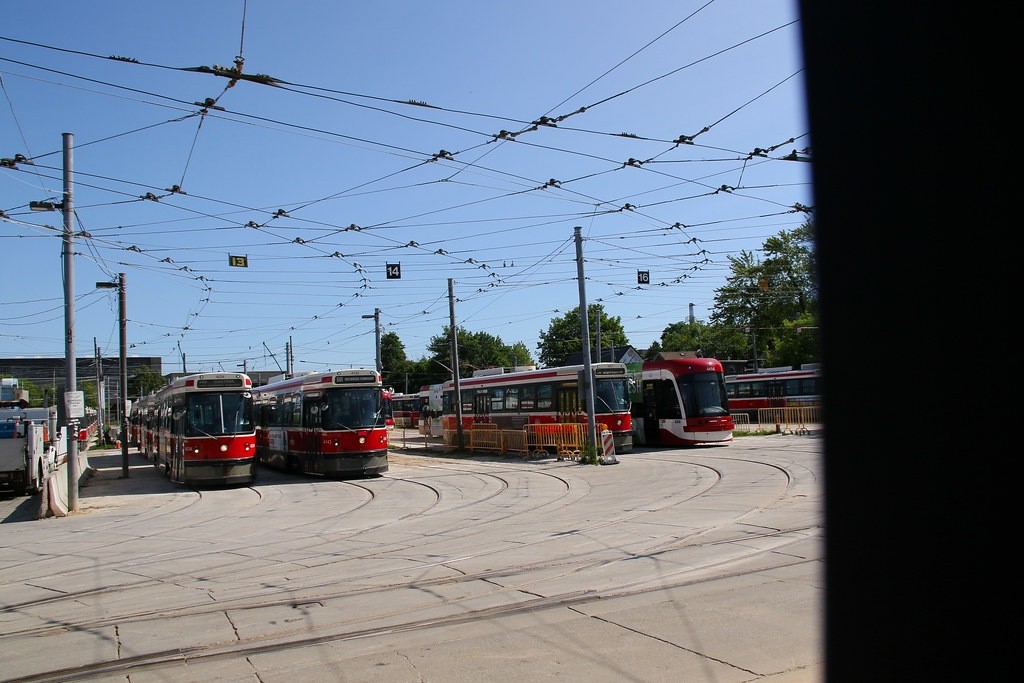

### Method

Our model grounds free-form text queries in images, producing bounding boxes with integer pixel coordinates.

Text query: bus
[724,367,821,421]
[394,357,734,455]
[196,368,393,477]
[129,368,257,487]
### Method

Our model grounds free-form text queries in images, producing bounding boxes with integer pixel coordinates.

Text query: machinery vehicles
[0,377,58,492]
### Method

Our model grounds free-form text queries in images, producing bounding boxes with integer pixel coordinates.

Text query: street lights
[29,201,79,512]
[95,281,129,479]
[362,312,380,378]
[238,364,247,373]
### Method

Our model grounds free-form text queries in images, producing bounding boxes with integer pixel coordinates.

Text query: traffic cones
[116,441,120,449]
